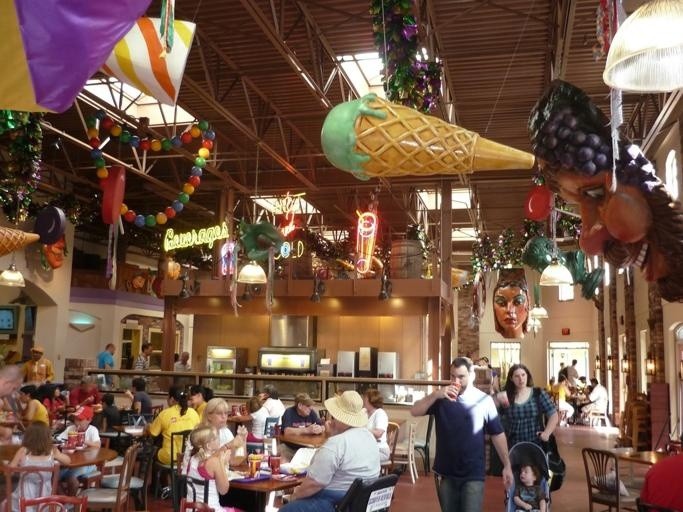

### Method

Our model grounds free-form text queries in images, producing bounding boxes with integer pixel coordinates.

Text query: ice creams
[321,93,536,182]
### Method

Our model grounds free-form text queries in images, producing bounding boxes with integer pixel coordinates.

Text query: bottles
[0,409,14,422]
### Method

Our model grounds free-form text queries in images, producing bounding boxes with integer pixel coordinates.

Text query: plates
[272,474,295,482]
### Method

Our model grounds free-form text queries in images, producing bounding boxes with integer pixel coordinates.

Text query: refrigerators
[204,345,247,395]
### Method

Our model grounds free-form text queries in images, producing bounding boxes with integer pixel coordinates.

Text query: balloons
[525,185,554,221]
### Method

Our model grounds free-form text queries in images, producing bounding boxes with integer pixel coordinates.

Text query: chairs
[2,406,434,511]
[544,383,613,431]
[580,444,638,512]
[632,495,671,512]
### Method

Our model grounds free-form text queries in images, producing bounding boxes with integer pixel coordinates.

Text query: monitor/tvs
[0,305,19,334]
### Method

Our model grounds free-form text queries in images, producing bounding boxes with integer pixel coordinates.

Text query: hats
[30,345,44,353]
[294,391,317,406]
[323,390,369,428]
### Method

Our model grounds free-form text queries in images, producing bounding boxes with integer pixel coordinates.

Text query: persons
[411,358,513,512]
[546,167,672,280]
[479,357,500,393]
[493,364,559,443]
[640,432,683,512]
[492,277,528,338]
[543,359,608,428]
[514,464,548,512]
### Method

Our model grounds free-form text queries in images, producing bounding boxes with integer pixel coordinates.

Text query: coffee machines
[241,365,257,397]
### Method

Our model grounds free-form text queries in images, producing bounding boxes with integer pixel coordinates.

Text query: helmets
[69,405,94,421]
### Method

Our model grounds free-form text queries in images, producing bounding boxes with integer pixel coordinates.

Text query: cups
[76,432,84,447]
[247,452,281,477]
[274,423,281,434]
[669,440,681,455]
[231,405,238,416]
[239,404,248,415]
[67,432,77,448]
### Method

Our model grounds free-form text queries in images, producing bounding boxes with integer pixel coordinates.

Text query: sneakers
[160,486,172,500]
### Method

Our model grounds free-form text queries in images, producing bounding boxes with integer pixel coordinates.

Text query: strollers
[503,431,553,512]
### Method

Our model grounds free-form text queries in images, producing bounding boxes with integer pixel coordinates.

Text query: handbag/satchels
[539,424,566,494]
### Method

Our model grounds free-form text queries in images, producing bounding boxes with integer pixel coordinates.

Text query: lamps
[589,350,657,377]
[537,209,574,288]
[526,302,548,329]
[377,273,392,300]
[309,276,326,305]
[240,283,261,300]
[235,143,269,286]
[0,247,28,288]
[601,0,682,94]
[177,276,201,299]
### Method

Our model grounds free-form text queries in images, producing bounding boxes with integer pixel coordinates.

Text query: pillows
[591,467,630,498]
